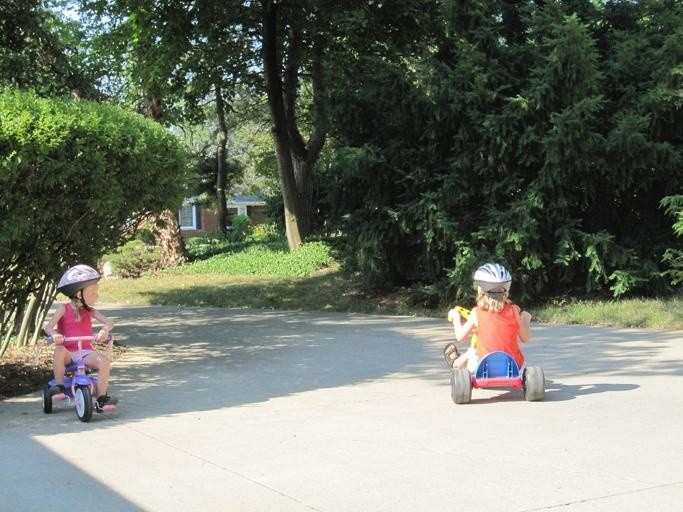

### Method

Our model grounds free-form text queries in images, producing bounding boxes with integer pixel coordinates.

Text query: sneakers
[96,391,119,413]
[47,384,66,396]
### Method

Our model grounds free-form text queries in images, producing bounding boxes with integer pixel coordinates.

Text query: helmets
[474,262,512,293]
[57,264,101,297]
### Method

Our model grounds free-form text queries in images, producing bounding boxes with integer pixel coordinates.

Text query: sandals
[443,343,461,369]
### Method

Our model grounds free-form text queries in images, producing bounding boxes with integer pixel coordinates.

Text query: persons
[443,262,533,378]
[41,264,121,412]
